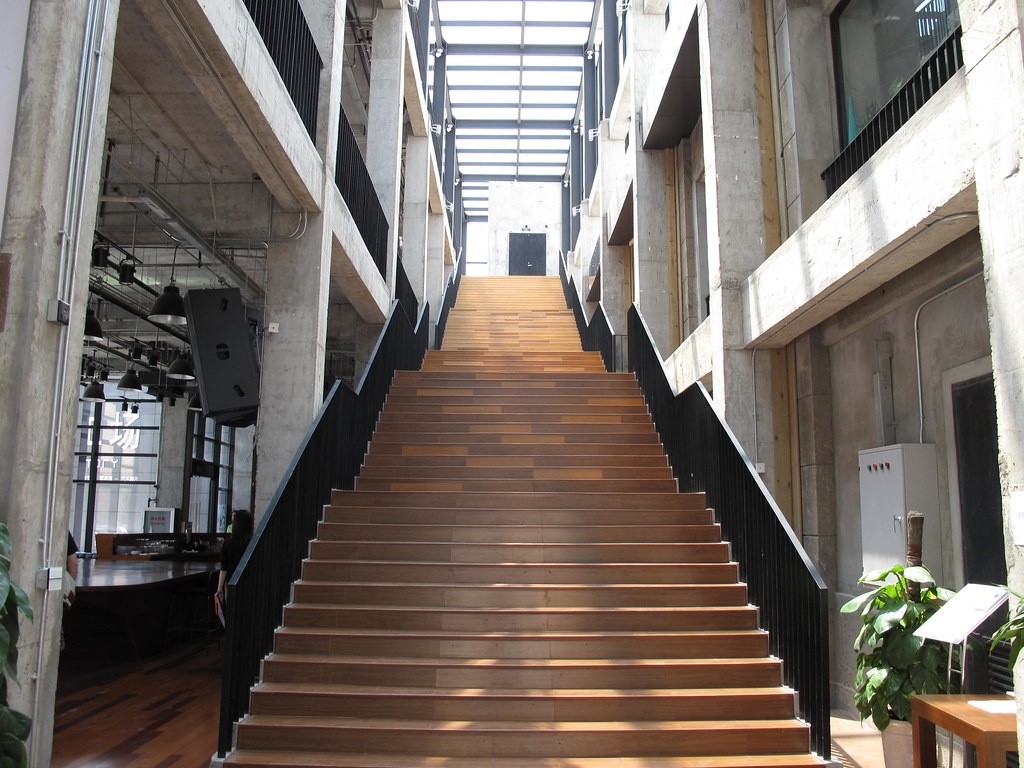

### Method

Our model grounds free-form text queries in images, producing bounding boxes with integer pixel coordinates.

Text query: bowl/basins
[117,546,129,553]
[76,552,97,559]
[128,546,137,553]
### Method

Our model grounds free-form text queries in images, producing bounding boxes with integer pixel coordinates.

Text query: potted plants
[840,559,956,768]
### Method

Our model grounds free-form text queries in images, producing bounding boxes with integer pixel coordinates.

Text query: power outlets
[46,298,71,326]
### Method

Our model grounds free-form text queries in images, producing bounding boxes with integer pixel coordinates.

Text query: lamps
[429,46,447,58]
[82,308,104,343]
[91,238,137,286]
[584,46,596,60]
[569,123,583,135]
[562,179,570,189]
[81,365,110,403]
[148,285,189,326]
[121,402,139,414]
[132,345,158,368]
[454,178,461,186]
[156,392,176,406]
[166,352,196,381]
[446,122,454,133]
[117,369,160,397]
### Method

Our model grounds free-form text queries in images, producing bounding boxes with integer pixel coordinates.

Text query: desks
[913,693,1019,768]
[60,558,222,667]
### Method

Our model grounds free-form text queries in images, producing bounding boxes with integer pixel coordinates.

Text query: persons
[180,527,195,552]
[226,510,238,533]
[215,509,255,605]
[66,530,78,581]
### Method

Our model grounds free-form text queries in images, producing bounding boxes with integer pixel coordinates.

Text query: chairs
[161,566,224,659]
